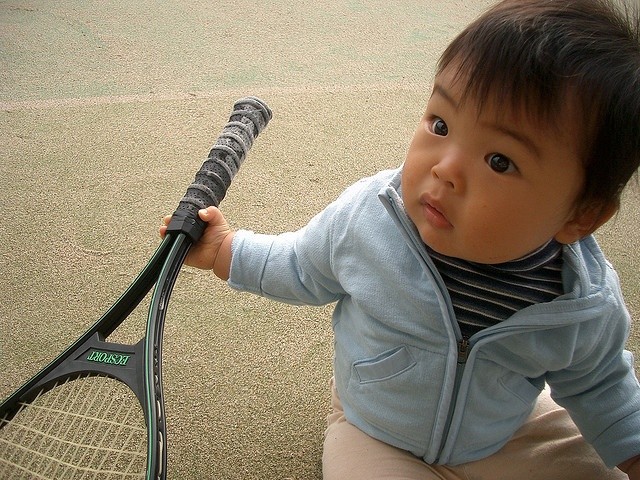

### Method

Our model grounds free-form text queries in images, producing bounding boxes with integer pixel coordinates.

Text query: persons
[158,0,640,478]
